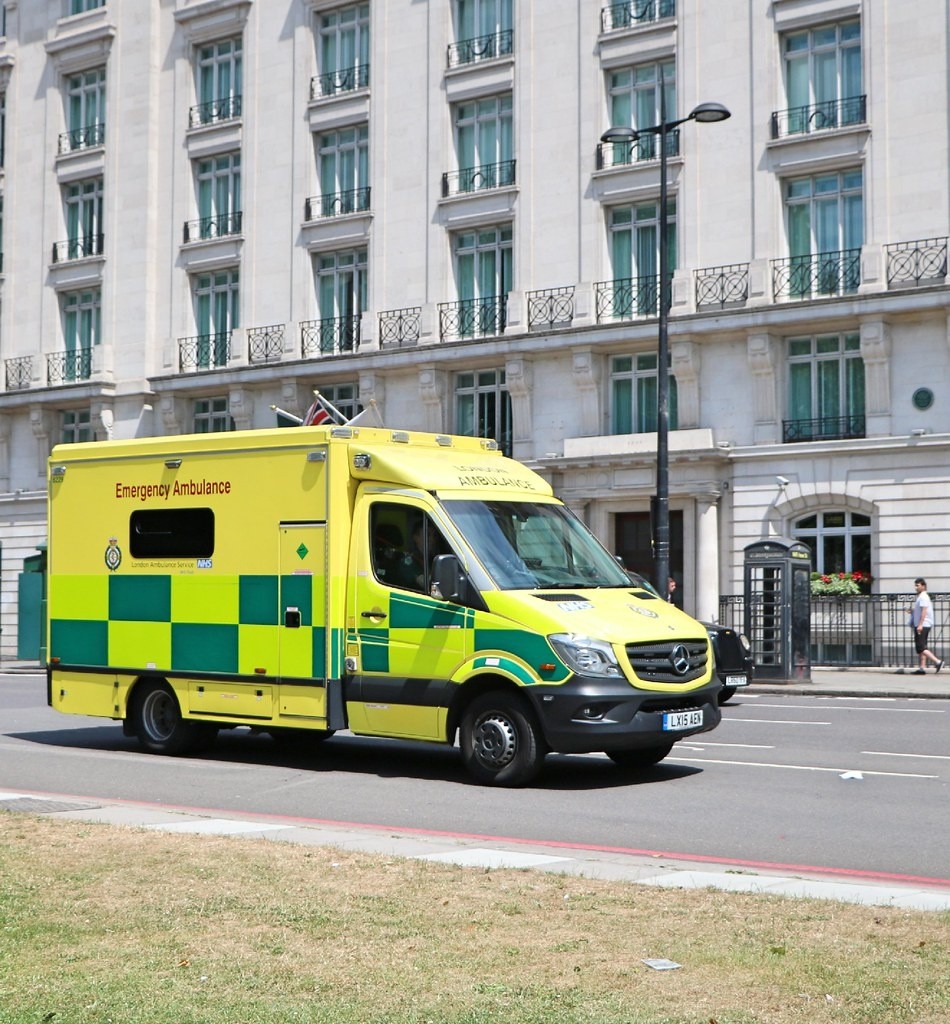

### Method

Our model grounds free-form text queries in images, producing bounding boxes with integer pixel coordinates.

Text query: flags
[302,400,335,426]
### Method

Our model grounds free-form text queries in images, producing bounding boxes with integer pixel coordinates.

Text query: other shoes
[935,660,946,674]
[910,670,926,675]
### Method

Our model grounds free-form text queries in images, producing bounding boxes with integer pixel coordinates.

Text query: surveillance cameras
[776,476,789,485]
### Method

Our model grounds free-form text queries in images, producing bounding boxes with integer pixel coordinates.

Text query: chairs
[373,524,404,586]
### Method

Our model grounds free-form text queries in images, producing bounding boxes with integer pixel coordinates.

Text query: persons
[391,520,436,591]
[667,578,677,604]
[905,578,945,675]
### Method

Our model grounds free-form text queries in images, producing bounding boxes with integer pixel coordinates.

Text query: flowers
[809,571,874,596]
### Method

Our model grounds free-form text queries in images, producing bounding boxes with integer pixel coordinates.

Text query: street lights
[602,99,730,602]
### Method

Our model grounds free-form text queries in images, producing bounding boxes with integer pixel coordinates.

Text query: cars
[626,570,754,708]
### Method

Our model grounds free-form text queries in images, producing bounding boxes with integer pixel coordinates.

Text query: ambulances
[46,419,723,786]
